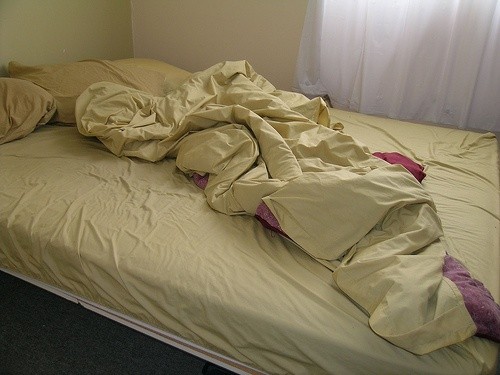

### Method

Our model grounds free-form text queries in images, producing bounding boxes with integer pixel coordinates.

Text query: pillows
[8,58,198,128]
[0,74,57,145]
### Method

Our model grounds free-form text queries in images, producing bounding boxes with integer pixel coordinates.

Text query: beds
[1,54,500,375]
